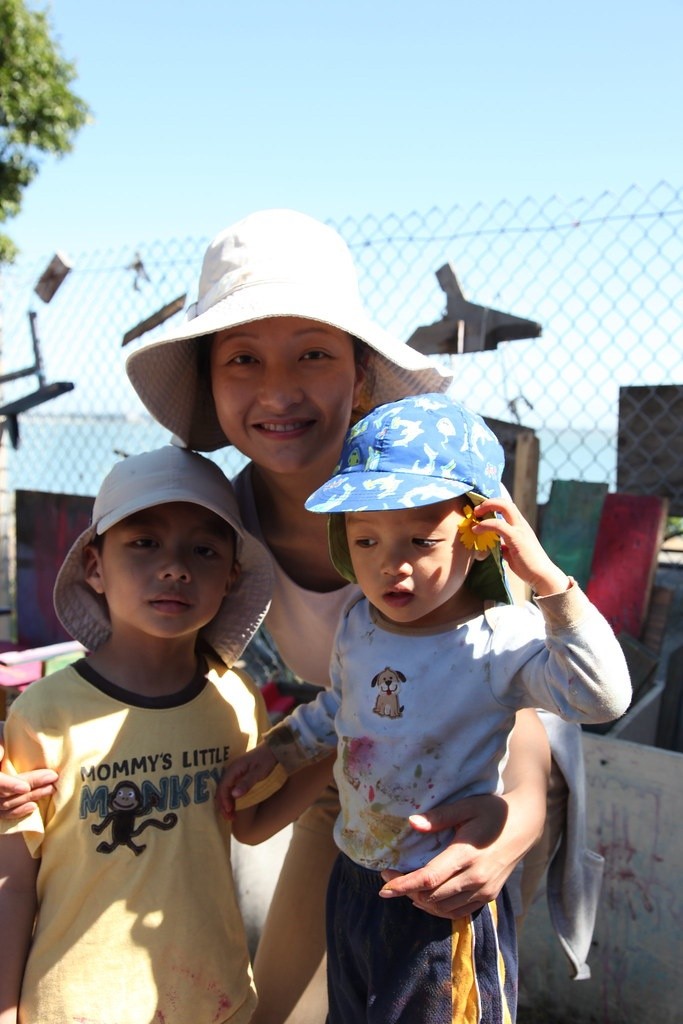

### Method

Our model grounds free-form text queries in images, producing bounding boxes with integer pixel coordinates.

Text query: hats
[304,393,514,606]
[126,210,454,452]
[52,445,274,669]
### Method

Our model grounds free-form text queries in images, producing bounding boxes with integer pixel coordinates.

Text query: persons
[0,210,606,1024]
[0,446,330,1023]
[215,395,632,1024]
